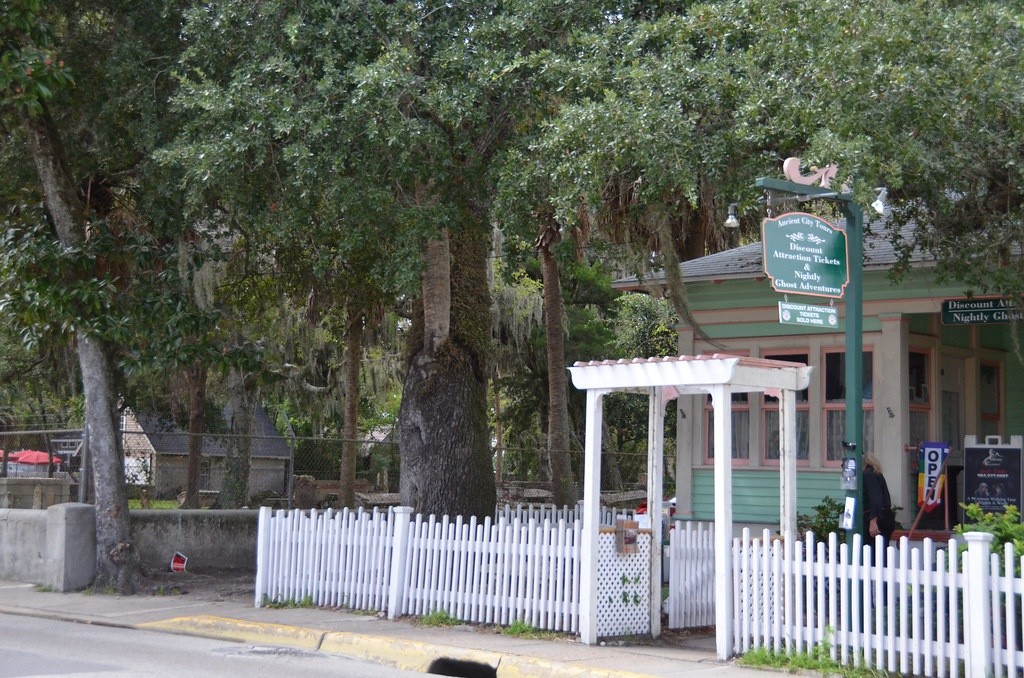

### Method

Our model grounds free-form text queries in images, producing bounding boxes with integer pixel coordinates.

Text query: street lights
[723,187,889,654]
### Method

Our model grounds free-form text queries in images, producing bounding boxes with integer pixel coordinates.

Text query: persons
[861,452,896,566]
[993,484,1008,498]
[985,449,1008,466]
[975,483,990,497]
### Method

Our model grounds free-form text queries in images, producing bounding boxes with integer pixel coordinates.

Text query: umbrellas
[0,449,61,477]
